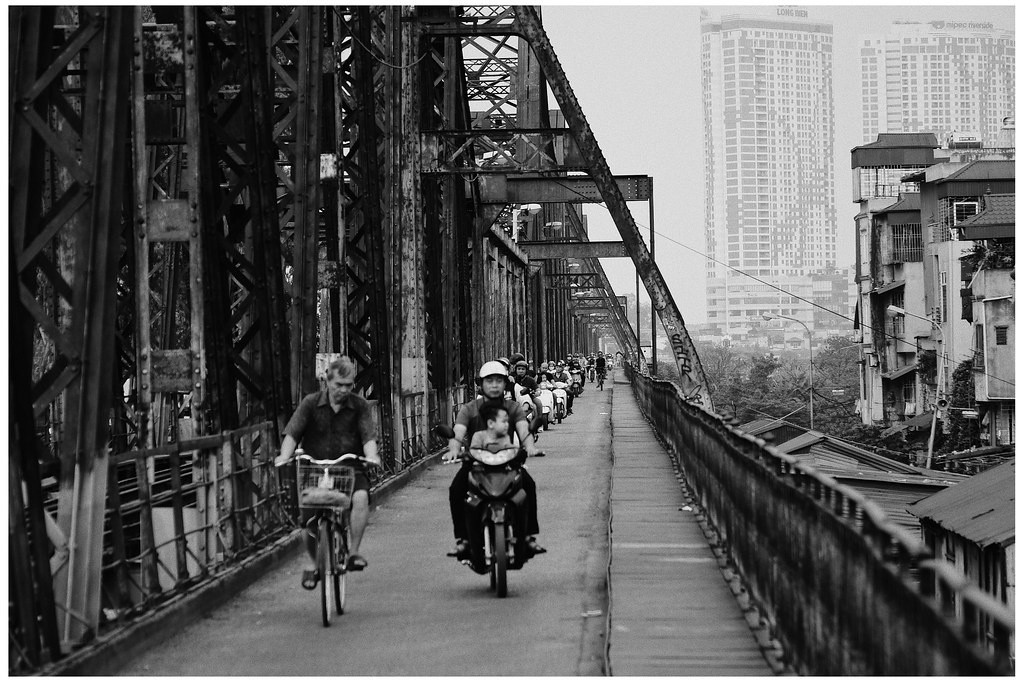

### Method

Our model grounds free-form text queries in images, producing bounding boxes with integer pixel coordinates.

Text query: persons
[439,360,548,557]
[469,401,521,467]
[496,351,615,442]
[274,357,383,591]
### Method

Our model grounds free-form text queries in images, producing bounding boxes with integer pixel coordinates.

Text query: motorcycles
[507,358,618,441]
[430,416,544,595]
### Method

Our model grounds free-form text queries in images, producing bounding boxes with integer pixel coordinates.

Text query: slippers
[302,568,317,590]
[349,554,368,567]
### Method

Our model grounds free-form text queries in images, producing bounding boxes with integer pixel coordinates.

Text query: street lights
[888,305,947,468]
[763,313,814,429]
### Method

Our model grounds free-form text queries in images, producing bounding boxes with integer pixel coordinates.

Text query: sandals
[446,535,549,558]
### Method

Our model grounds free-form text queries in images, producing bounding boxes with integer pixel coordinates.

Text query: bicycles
[274,447,376,629]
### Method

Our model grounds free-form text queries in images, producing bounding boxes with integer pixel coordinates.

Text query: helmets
[495,357,510,370]
[479,360,507,378]
[515,361,528,371]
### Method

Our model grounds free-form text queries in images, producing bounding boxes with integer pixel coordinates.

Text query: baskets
[296,465,356,510]
